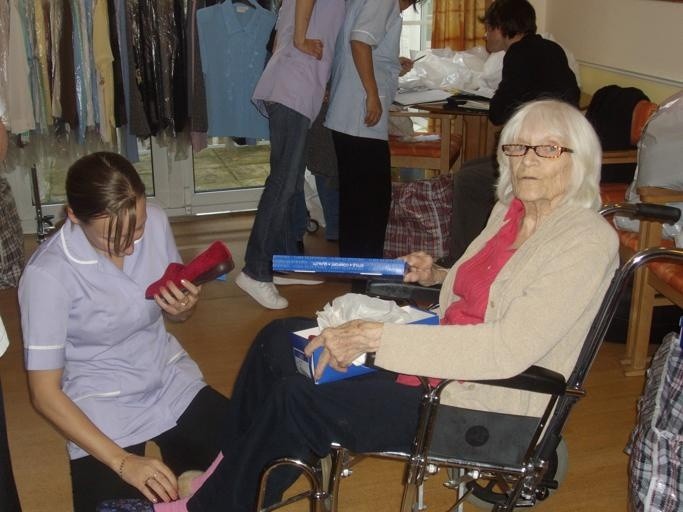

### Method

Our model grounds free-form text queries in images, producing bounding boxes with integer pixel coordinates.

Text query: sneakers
[235,270,291,311]
[96,496,155,512]
[273,274,326,287]
[144,240,236,300]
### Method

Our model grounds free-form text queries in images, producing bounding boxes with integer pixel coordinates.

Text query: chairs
[385,108,466,177]
[579,84,683,378]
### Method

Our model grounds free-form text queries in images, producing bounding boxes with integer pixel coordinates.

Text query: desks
[410,92,593,183]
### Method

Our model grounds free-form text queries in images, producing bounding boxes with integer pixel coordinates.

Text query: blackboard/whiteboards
[544,0,683,88]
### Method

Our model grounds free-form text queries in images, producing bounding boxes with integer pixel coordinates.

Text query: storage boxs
[287,305,440,386]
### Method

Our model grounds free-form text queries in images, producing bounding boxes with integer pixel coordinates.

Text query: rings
[181,298,190,307]
[145,478,155,485]
[153,470,159,480]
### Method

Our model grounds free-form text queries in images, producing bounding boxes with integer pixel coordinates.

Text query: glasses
[500,141,576,158]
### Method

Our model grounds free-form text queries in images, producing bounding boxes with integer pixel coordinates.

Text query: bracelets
[119,452,133,478]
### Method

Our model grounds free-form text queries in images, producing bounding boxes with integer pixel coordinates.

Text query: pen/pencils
[410,55,426,64]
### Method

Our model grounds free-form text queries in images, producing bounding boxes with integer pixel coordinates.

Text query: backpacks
[585,83,653,185]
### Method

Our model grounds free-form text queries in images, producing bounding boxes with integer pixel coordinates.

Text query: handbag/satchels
[1,176,27,293]
[622,318,683,510]
[381,174,454,267]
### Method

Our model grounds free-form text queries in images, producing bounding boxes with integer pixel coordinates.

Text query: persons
[322,1,427,260]
[94,96,621,512]
[435,0,581,269]
[0,116,26,291]
[266,22,341,256]
[15,149,228,511]
[234,0,346,311]
